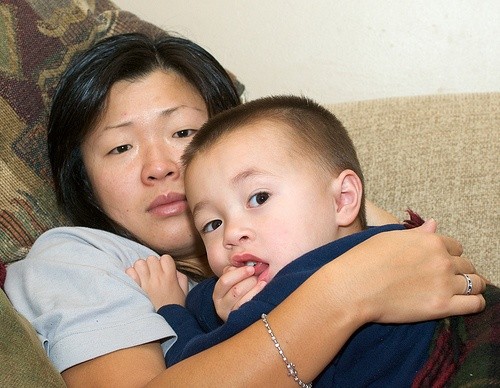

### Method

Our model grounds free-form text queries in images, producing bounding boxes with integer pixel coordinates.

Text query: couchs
[0,1,500,388]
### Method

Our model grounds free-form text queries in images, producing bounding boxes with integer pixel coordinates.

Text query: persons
[128,93,500,388]
[0,28,490,388]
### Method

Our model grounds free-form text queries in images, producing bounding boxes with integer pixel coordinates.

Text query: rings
[461,272,472,295]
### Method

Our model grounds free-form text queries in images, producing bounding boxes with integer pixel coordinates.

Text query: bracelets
[261,314,314,388]
